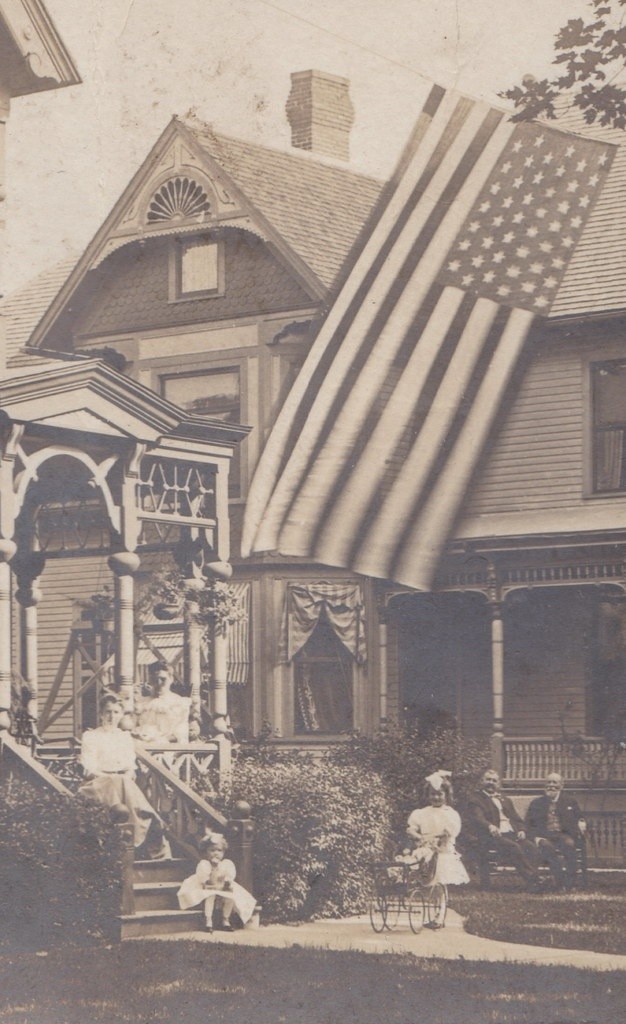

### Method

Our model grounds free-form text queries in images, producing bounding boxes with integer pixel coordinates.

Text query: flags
[239,85,619,592]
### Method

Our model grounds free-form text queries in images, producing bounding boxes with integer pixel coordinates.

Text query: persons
[136,662,192,742]
[470,770,586,893]
[78,694,173,861]
[177,835,257,932]
[408,773,470,930]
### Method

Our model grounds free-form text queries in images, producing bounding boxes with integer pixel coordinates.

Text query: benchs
[486,797,588,877]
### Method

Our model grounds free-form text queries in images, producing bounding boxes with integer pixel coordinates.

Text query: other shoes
[205,926,213,932]
[424,922,444,929]
[220,922,235,931]
